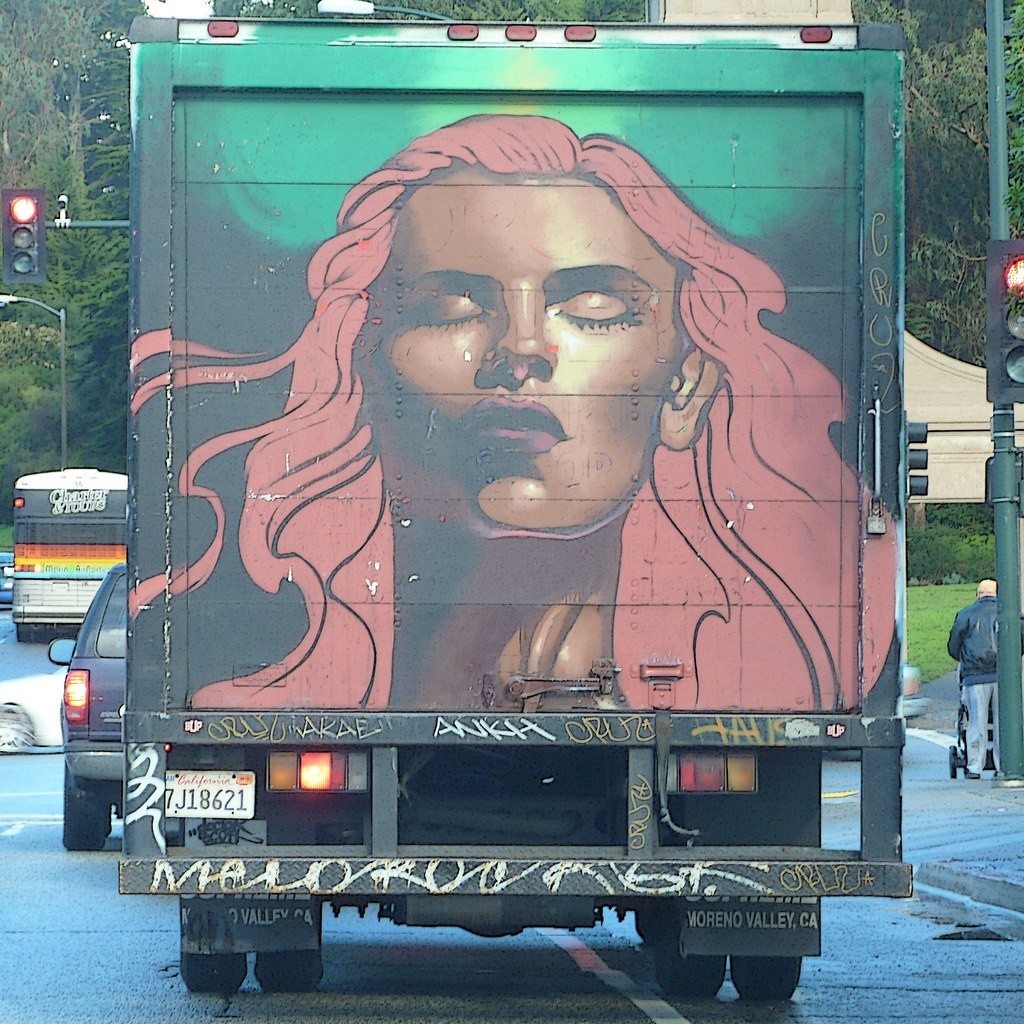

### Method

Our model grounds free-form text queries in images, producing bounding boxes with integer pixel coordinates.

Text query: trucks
[122,15,914,1001]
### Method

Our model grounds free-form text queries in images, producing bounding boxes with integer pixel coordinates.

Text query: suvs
[48,564,127,850]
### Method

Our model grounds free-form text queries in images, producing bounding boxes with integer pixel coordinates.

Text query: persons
[948,579,1024,778]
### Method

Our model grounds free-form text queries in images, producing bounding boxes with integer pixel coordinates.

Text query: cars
[901,664,928,719]
[0,554,14,604]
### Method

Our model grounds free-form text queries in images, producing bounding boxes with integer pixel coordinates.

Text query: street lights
[0,294,68,471]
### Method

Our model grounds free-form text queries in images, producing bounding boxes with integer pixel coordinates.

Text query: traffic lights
[0,189,46,288]
[985,242,1024,404]
[903,414,930,502]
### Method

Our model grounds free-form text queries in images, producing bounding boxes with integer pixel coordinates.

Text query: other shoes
[990,771,998,780]
[965,772,980,779]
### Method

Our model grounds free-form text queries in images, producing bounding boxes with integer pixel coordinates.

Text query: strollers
[950,659,999,781]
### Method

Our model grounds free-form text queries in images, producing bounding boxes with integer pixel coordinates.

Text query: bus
[12,468,129,641]
